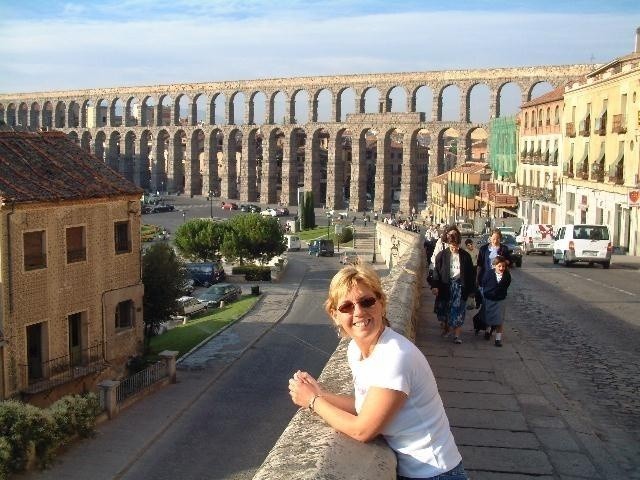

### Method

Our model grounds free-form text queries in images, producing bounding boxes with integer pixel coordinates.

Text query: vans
[514,224,552,255]
[179,261,224,287]
[281,234,301,252]
[552,224,612,269]
[308,239,334,257]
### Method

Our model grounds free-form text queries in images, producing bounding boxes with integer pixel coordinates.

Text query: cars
[197,282,242,307]
[496,227,515,238]
[457,223,475,238]
[339,251,358,265]
[141,193,175,213]
[220,201,289,216]
[476,234,523,267]
[171,296,205,317]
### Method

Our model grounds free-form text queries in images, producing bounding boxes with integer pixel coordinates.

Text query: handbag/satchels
[427,269,433,284]
[473,312,494,331]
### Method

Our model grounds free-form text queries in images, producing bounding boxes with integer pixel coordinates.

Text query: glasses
[333,295,380,314]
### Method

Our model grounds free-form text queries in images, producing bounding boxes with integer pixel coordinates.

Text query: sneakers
[453,337,462,343]
[494,339,502,347]
[484,332,490,340]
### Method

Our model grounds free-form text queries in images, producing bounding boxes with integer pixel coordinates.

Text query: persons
[483,256,512,347]
[285,262,471,479]
[314,188,511,311]
[431,230,473,344]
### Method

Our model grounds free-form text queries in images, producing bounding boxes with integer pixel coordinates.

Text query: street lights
[207,190,216,219]
[161,181,164,194]
[158,227,171,241]
[181,212,186,223]
[372,218,377,263]
[326,212,331,240]
[351,223,356,250]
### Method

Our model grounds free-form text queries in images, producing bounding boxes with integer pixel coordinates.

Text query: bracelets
[308,393,323,414]
